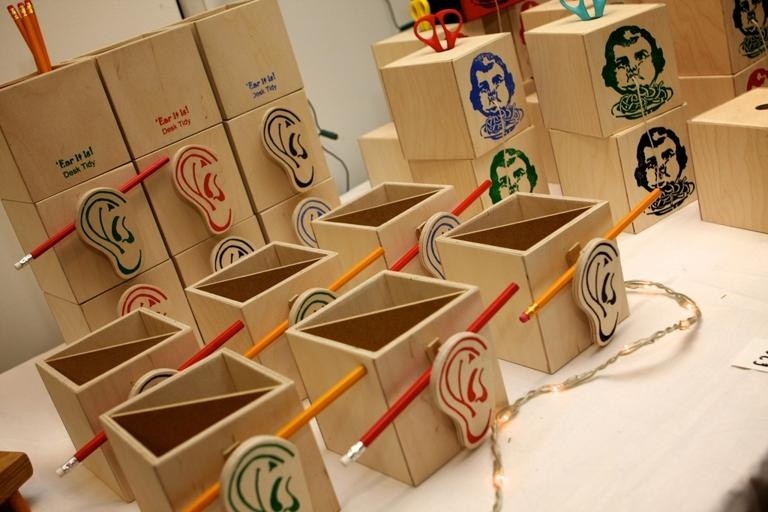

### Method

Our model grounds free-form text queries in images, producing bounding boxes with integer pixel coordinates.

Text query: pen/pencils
[241,246,382,360]
[518,186,663,322]
[185,364,367,512]
[387,181,494,271]
[12,154,172,271]
[7,0,53,73]
[340,282,520,468]
[54,319,244,478]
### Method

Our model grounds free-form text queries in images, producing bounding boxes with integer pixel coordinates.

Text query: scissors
[408,0,463,51]
[560,0,607,21]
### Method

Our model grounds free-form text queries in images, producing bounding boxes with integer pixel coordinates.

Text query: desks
[0,180,768,512]
